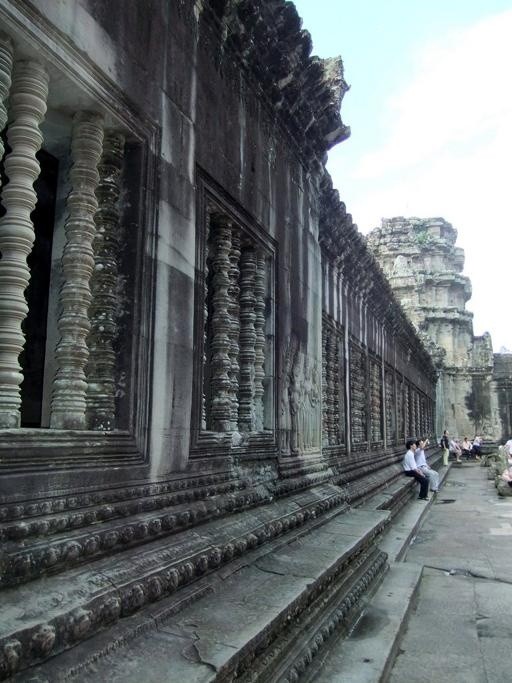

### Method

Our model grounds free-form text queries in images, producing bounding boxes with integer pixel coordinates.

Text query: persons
[415,439,440,493]
[449,434,482,463]
[403,440,428,500]
[440,430,449,465]
